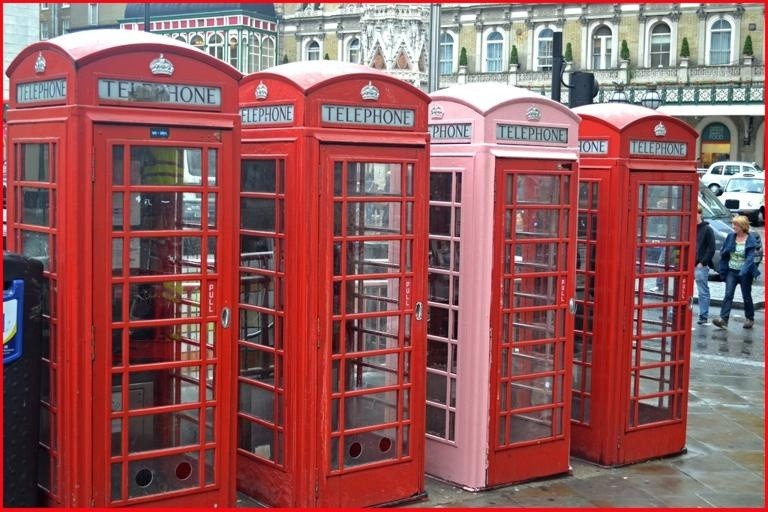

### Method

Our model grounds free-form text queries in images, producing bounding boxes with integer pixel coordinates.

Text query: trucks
[113,149,217,241]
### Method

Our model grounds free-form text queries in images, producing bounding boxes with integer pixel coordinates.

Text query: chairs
[111,268,176,447]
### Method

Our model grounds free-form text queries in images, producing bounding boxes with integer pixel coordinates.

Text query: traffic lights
[567,72,599,106]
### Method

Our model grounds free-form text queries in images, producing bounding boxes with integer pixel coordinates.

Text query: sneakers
[697,318,707,324]
[713,318,728,330]
[743,319,754,328]
[650,287,663,292]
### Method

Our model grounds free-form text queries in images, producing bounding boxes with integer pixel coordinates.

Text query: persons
[649,199,684,292]
[712,215,763,331]
[660,204,717,321]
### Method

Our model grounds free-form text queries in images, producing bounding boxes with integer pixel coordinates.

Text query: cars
[702,160,761,194]
[720,171,765,222]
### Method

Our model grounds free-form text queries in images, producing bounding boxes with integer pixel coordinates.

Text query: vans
[640,177,763,275]
